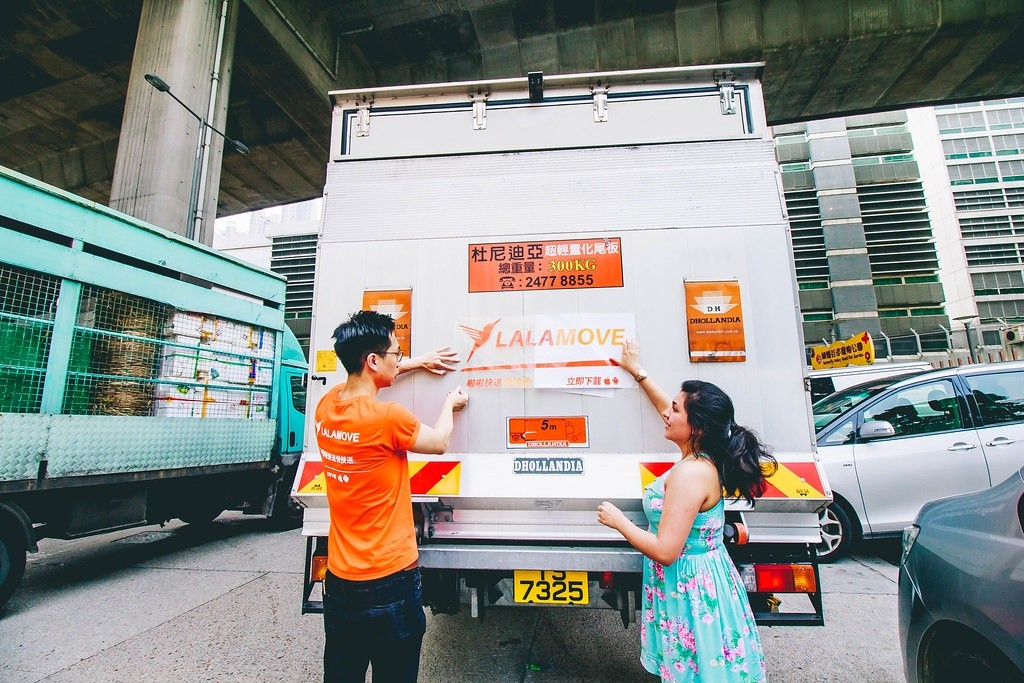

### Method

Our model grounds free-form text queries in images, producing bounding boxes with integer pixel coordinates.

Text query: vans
[807,361,949,414]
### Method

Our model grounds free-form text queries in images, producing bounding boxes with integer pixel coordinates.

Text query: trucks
[291,61,833,626]
[0,169,306,615]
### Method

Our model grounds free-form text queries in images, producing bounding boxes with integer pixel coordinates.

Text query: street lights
[144,72,250,241]
[951,314,982,364]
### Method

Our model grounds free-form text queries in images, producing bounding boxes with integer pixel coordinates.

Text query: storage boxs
[153,307,275,418]
[0,321,94,414]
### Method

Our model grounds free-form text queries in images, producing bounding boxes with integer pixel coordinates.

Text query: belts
[397,560,420,572]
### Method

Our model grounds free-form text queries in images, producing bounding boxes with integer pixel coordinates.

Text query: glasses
[366,349,405,362]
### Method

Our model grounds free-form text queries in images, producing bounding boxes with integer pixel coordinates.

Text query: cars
[897,467,1024,683]
[808,360,1024,562]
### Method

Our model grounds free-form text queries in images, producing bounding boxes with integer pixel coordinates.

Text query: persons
[595,335,778,683]
[314,310,468,683]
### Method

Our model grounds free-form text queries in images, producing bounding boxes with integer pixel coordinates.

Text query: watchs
[634,367,648,383]
